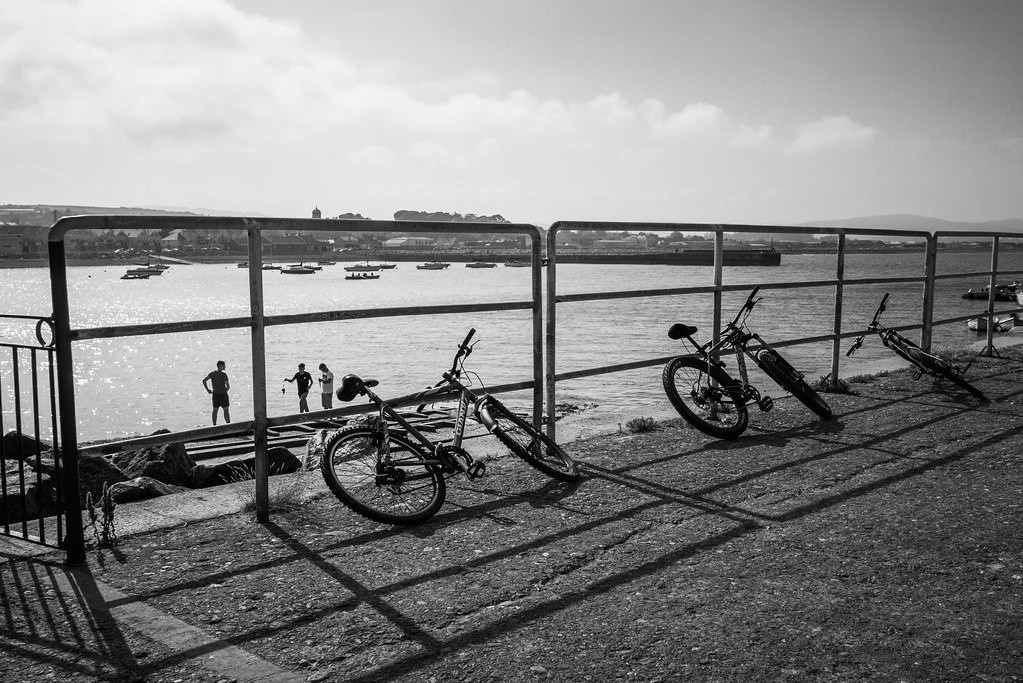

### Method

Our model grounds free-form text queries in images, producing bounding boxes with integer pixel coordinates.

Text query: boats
[318,258,337,265]
[237,261,250,269]
[282,259,322,274]
[504,258,532,267]
[262,263,280,270]
[378,258,397,270]
[343,259,382,272]
[465,261,496,268]
[998,316,1015,332]
[962,280,1023,307]
[121,262,169,280]
[345,270,381,280]
[967,317,988,331]
[416,261,451,270]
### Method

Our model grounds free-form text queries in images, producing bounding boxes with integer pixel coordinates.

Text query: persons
[202,360,230,427]
[284,363,313,413]
[317,363,333,410]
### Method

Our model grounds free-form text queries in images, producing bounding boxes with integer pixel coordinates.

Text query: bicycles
[317,328,583,529]
[846,293,985,402]
[661,285,833,438]
